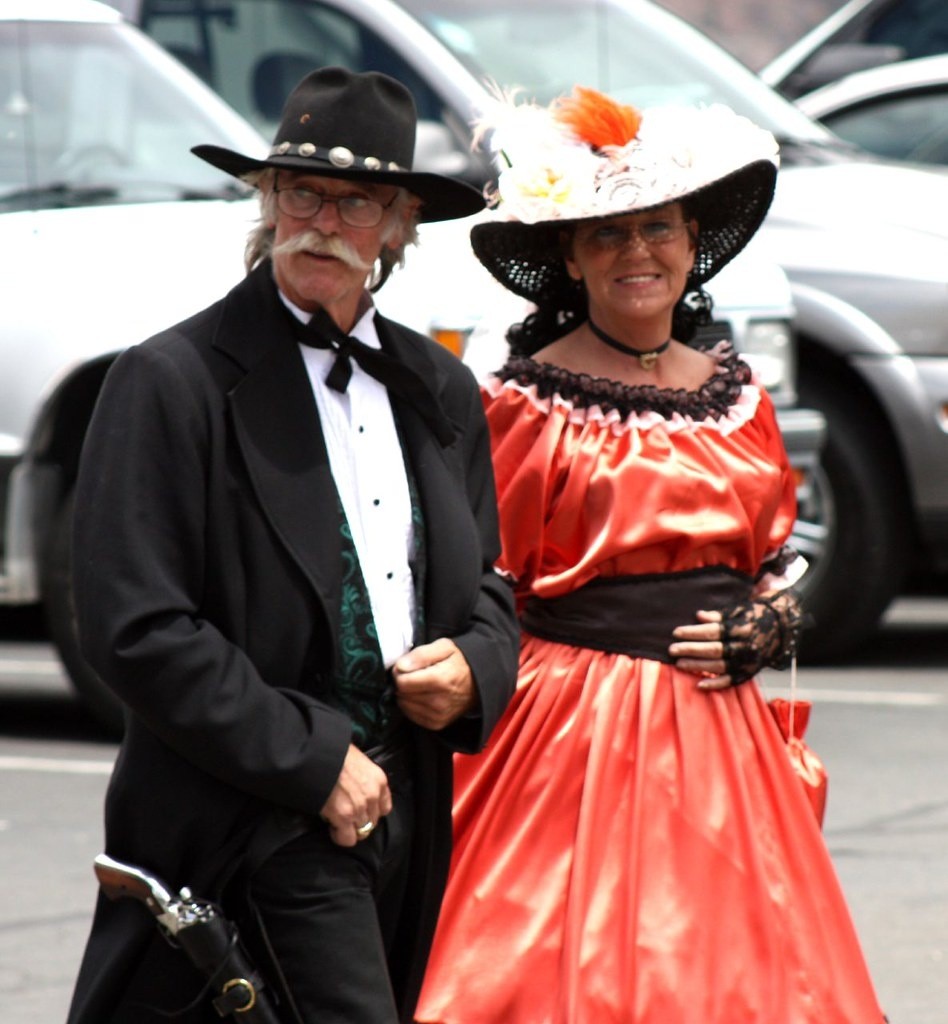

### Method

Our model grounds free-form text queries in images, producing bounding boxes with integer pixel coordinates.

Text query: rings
[357,822,374,836]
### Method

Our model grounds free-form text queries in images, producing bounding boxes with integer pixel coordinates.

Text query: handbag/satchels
[765,698,828,831]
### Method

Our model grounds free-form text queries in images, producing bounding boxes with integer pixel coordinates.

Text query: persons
[412,92,889,1024]
[69,69,522,1024]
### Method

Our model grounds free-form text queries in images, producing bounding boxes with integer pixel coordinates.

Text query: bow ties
[284,301,457,452]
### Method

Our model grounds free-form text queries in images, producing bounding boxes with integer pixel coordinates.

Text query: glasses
[571,218,692,250]
[273,167,400,228]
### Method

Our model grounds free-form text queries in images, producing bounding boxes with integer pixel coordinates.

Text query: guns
[93,853,215,935]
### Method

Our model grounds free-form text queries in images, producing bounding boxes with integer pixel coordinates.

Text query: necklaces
[587,319,668,370]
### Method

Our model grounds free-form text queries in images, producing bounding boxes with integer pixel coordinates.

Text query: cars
[2,1,948,743]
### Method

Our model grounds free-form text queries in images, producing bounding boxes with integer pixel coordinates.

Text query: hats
[471,85,781,308]
[190,68,486,224]
